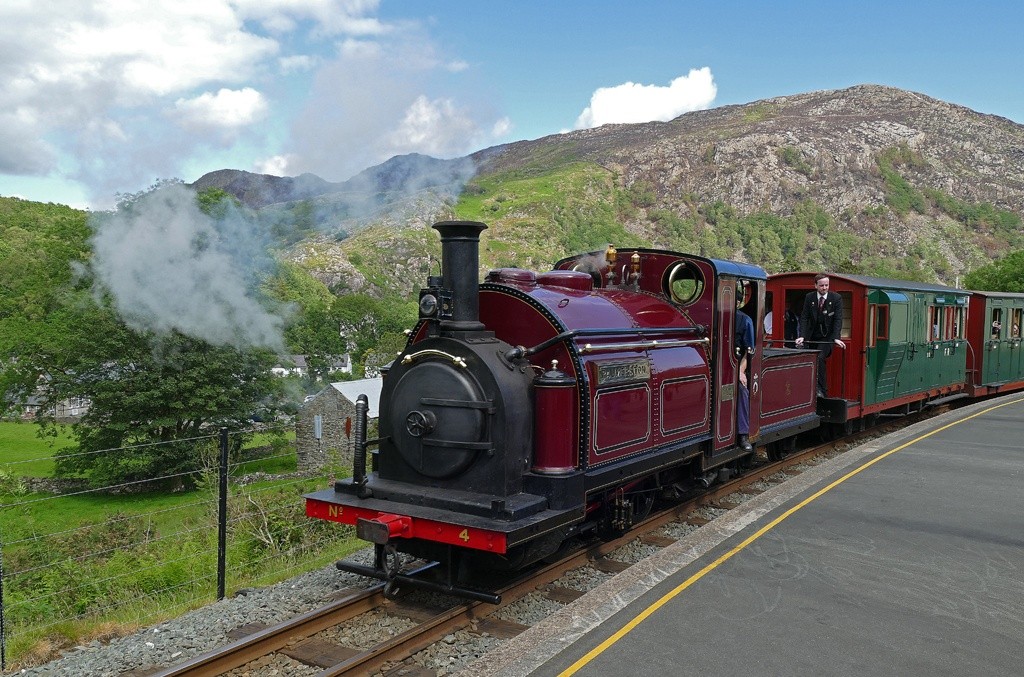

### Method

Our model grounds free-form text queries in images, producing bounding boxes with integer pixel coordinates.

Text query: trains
[298,219,1023,604]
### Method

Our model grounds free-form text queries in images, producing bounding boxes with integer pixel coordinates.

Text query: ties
[818,297,824,313]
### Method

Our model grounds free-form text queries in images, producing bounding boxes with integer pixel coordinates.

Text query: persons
[795,274,847,398]
[734,288,755,452]
[992,322,1002,339]
[764,293,801,349]
[1013,323,1020,335]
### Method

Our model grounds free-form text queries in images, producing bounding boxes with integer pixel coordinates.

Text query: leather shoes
[738,435,752,452]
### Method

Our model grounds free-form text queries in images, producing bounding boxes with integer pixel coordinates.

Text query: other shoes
[816,390,825,399]
